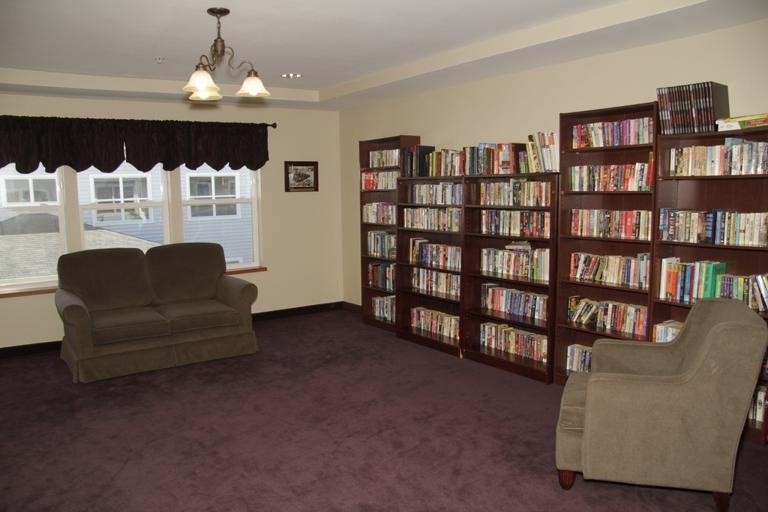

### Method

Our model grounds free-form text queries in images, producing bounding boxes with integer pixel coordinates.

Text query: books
[567,153,653,374]
[572,117,653,149]
[405,131,559,179]
[362,149,402,322]
[654,138,768,422]
[403,181,462,341]
[716,113,768,131]
[481,179,551,365]
[657,80,730,136]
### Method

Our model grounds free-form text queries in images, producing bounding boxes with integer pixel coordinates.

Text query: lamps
[182,7,271,101]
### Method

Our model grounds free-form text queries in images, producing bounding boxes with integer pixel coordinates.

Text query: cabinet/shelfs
[553,100,658,392]
[391,171,558,386]
[358,134,421,333]
[648,125,768,449]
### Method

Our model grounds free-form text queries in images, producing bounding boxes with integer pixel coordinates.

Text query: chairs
[556,297,767,512]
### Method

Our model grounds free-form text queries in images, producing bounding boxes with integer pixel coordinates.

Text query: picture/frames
[284,161,318,192]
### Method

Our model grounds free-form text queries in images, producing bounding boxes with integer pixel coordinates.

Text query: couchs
[55,243,258,385]
